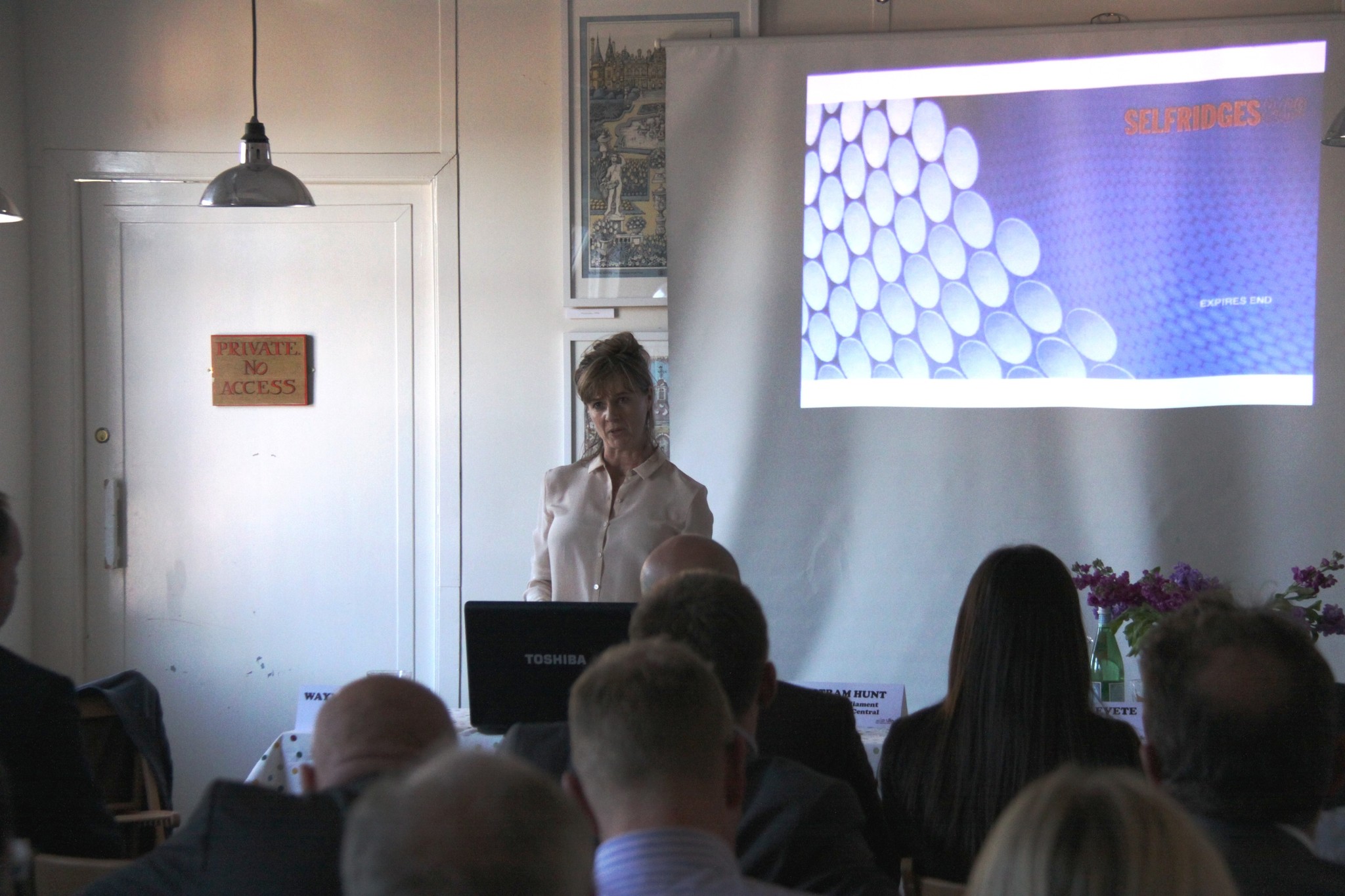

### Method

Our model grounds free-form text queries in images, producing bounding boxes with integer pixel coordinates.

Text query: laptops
[463,601,640,734]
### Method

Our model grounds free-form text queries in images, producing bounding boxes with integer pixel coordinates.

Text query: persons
[522,331,714,604]
[0,516,1345,896]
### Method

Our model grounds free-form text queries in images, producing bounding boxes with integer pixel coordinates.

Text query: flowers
[1069,551,1345,657]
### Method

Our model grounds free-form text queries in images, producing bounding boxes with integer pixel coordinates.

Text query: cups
[1132,679,1145,702]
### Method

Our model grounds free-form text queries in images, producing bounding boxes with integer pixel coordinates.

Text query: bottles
[1090,603,1126,704]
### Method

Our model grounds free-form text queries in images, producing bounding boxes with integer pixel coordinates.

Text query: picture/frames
[561,0,758,306]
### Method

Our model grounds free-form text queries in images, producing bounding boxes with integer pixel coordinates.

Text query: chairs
[78,670,180,863]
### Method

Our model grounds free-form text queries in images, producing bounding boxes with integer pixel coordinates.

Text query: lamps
[198,0,316,207]
